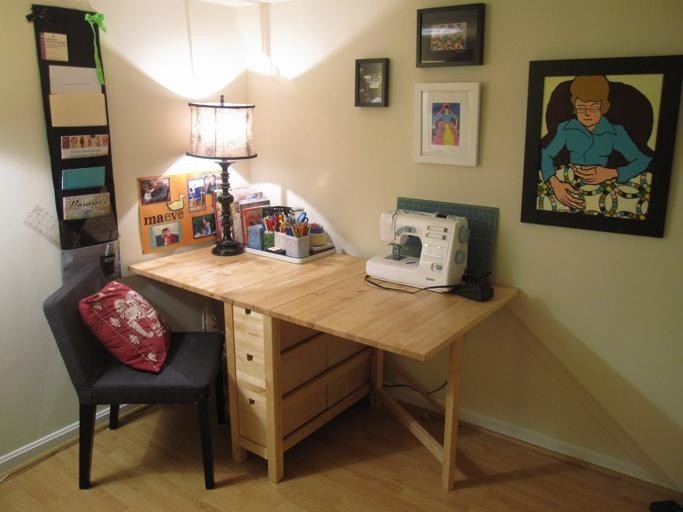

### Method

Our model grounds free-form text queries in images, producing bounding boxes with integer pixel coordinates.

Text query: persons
[540,74,654,209]
[431,22,466,51]
[194,220,211,239]
[431,103,459,145]
[188,175,216,209]
[144,181,168,205]
[162,228,177,247]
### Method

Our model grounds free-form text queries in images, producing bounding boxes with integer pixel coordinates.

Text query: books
[49,93,108,127]
[61,134,108,160]
[61,166,105,190]
[40,32,68,61]
[63,192,111,221]
[215,189,291,245]
[49,65,103,94]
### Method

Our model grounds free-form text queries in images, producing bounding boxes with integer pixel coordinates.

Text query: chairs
[43,265,226,491]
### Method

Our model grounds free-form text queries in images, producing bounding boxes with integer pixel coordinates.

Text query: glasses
[573,105,600,113]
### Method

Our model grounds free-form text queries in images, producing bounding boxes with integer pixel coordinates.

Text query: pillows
[79,279,171,373]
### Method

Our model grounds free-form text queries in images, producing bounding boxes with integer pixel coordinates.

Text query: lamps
[185,95,258,257]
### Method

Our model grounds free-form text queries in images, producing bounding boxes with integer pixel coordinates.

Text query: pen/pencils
[262,213,324,238]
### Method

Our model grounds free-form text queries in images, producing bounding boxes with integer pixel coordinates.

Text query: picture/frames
[415,3,486,68]
[520,54,683,238]
[413,81,482,168]
[354,56,390,108]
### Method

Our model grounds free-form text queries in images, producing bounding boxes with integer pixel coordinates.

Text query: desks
[128,235,520,492]
